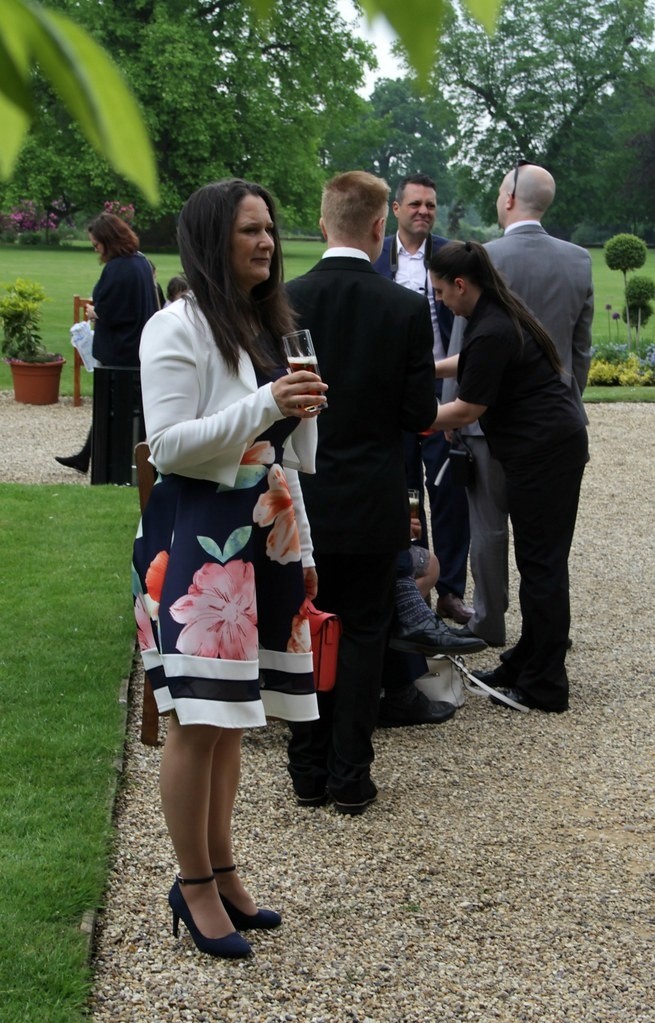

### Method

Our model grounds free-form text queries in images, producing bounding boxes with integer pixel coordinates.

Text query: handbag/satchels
[306,596,343,690]
[414,657,466,708]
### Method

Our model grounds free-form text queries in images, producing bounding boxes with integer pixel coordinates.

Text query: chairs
[72,294,95,407]
[134,437,159,748]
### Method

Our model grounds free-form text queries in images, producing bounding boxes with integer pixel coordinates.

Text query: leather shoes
[435,593,476,622]
[390,613,482,654]
[378,689,456,724]
[471,664,506,685]
[489,690,565,712]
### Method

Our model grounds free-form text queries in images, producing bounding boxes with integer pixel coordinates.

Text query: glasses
[512,159,541,199]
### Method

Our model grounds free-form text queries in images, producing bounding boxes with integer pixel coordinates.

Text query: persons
[439,162,596,646]
[282,171,438,815]
[374,174,475,626]
[373,518,488,726]
[56,214,189,484]
[429,240,590,712]
[130,178,329,956]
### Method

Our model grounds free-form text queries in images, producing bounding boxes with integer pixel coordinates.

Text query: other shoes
[55,455,89,475]
[335,779,377,814]
[451,625,505,647]
[296,783,329,807]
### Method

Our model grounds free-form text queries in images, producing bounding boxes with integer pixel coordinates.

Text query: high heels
[211,864,281,930]
[168,874,251,956]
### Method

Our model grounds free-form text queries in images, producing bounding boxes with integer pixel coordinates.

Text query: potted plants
[0,276,67,406]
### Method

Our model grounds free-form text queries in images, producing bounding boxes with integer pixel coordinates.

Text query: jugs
[69,318,100,372]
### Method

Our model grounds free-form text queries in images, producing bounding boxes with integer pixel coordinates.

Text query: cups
[282,328,328,412]
[407,489,420,542]
